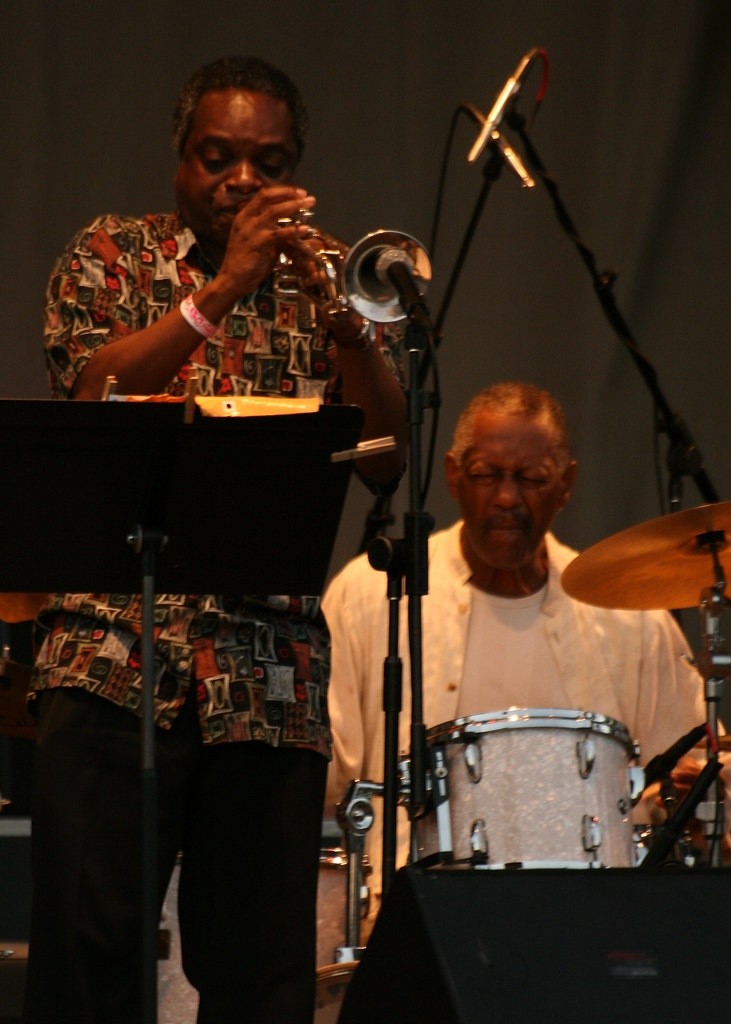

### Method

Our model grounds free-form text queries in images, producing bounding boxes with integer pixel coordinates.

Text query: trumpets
[241,199,433,324]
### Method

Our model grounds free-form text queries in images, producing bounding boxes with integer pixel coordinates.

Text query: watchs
[333,317,376,351]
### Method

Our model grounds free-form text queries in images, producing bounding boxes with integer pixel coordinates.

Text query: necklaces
[199,249,258,314]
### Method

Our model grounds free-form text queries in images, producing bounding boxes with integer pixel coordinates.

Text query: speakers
[337,867,731,1024]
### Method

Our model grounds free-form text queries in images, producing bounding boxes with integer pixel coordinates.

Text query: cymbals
[558,498,731,611]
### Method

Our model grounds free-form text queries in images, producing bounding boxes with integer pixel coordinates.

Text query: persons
[22,56,411,1024]
[318,381,731,922]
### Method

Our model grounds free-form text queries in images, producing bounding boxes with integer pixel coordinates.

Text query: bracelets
[180,295,219,338]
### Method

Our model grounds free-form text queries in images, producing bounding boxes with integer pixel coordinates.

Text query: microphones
[462,104,537,189]
[373,245,432,335]
[628,725,709,809]
[466,48,539,167]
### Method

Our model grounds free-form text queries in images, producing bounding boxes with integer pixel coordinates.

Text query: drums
[401,706,654,873]
